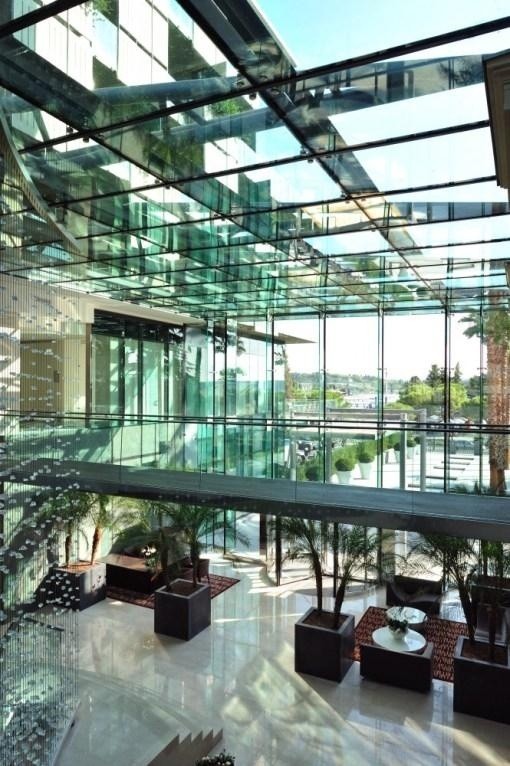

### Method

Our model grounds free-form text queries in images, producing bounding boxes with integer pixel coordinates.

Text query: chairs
[188,558,210,583]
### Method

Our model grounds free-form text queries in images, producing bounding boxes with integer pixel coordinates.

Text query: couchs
[386,574,444,614]
[360,640,435,692]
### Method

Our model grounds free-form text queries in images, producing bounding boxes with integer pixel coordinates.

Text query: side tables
[372,625,427,653]
[386,606,428,629]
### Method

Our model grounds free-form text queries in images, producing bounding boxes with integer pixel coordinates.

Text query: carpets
[105,569,242,612]
[353,606,469,682]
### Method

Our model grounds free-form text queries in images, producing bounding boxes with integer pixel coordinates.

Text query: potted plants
[386,617,408,640]
[404,482,510,726]
[147,501,251,643]
[334,435,421,484]
[47,485,149,611]
[266,507,355,684]
